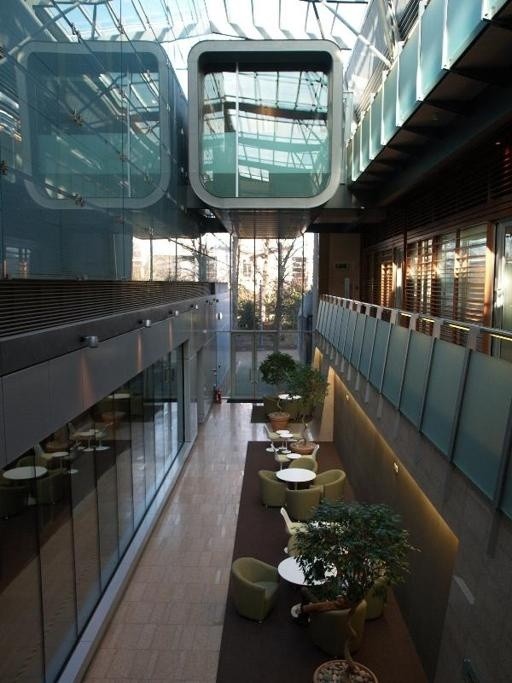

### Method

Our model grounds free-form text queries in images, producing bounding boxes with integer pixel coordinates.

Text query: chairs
[232,556,281,623]
[260,390,348,520]
[279,506,311,537]
[0,390,140,520]
[308,573,390,657]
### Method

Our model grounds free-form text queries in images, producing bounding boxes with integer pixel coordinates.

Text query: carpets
[250,404,271,422]
[0,437,132,594]
[122,404,165,422]
[212,441,429,682]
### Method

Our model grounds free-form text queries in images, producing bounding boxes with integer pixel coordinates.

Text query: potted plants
[295,497,420,683]
[279,366,330,455]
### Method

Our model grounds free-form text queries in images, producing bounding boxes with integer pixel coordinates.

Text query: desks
[277,555,338,626]
